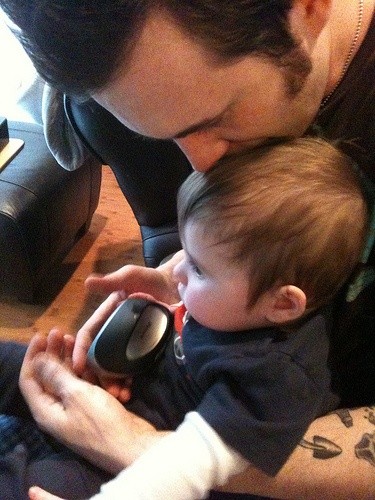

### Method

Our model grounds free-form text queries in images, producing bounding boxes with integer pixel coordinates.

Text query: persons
[0,0,375,500]
[0,135,371,500]
